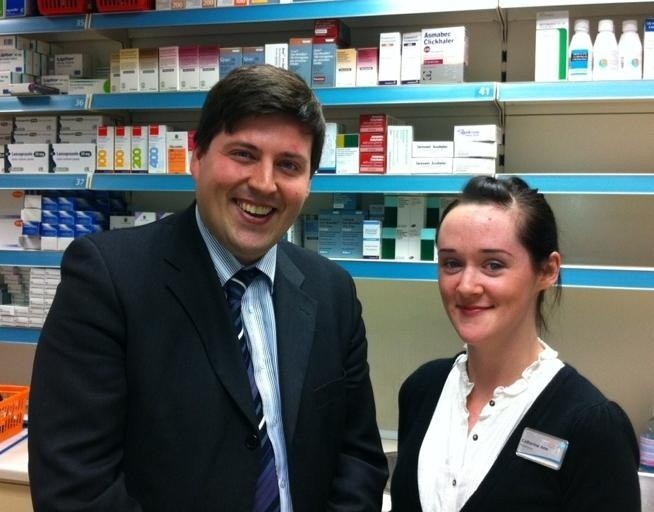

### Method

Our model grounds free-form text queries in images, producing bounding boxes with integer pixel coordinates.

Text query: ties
[223,269,280,512]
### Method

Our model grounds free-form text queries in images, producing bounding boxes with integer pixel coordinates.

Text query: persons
[391,174,641,512]
[28,65,389,511]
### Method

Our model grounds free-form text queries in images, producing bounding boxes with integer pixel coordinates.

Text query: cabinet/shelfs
[0,0,654,344]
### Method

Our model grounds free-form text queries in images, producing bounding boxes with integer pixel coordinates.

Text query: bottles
[569,17,641,81]
[639,417,654,471]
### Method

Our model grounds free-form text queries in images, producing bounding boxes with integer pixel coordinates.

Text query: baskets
[36,1,152,15]
[0,385,31,442]
